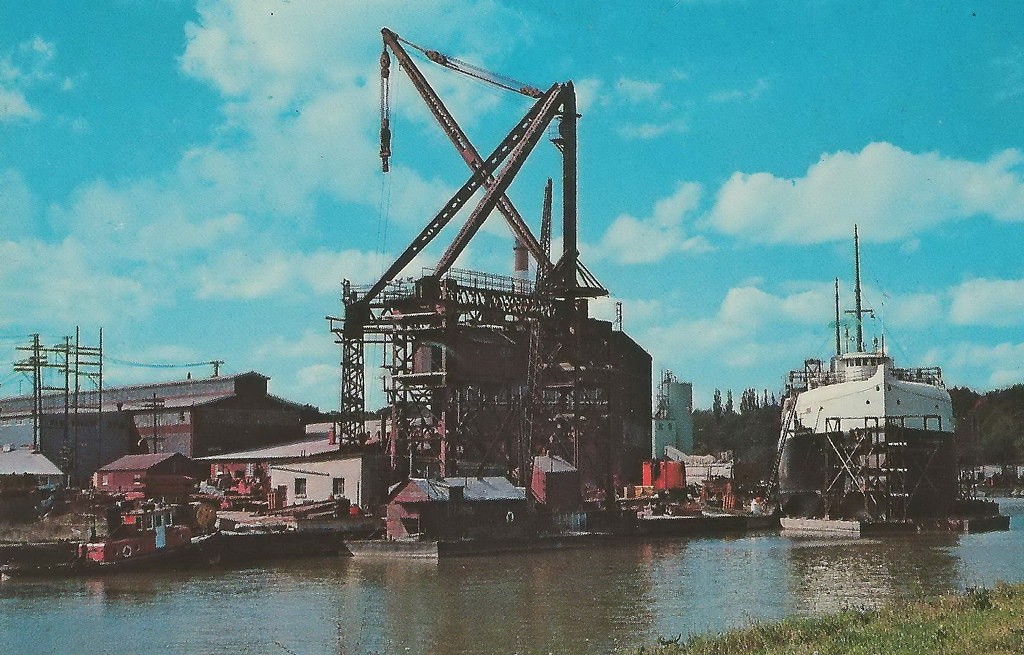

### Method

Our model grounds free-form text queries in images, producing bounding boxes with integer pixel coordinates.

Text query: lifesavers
[506,512,515,523]
[122,545,132,558]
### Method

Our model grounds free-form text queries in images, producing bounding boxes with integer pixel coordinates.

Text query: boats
[70,502,192,569]
[776,223,1012,537]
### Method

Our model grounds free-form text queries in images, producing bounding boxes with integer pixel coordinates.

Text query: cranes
[338,27,615,479]
